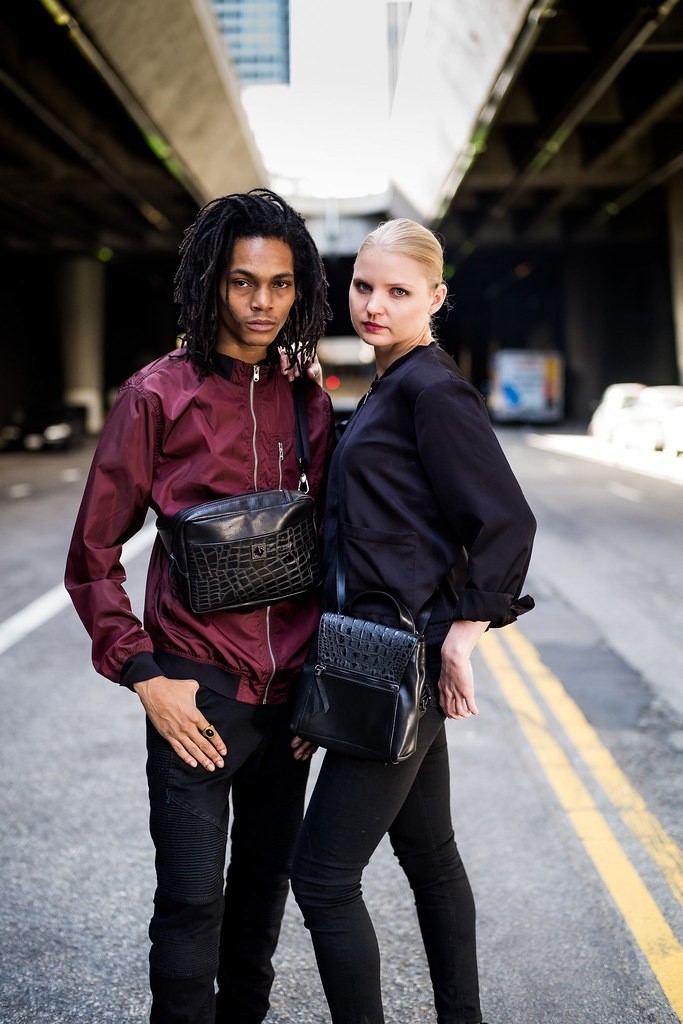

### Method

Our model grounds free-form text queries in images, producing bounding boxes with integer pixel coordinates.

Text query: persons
[64,184,537,1023]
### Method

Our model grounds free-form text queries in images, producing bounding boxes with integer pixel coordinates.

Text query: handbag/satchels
[290,590,432,765]
[151,490,322,615]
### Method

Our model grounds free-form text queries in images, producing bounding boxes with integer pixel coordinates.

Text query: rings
[202,725,216,739]
